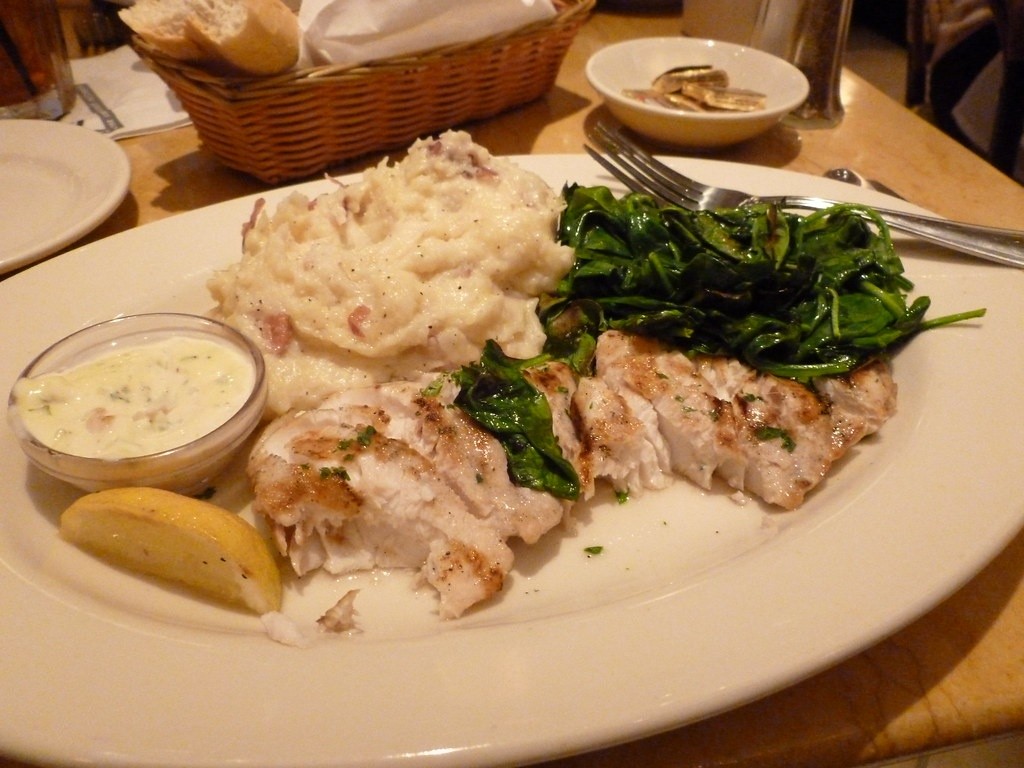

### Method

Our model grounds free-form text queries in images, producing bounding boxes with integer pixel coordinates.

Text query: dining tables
[0,0,1024,768]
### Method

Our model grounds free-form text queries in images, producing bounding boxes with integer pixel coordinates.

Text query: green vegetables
[449,183,987,502]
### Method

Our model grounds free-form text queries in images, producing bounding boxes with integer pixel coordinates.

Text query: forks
[584,121,1024,269]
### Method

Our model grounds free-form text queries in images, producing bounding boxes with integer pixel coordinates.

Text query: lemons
[58,487,280,617]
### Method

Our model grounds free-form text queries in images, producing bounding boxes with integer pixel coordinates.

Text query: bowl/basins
[7,312,269,495]
[586,36,809,147]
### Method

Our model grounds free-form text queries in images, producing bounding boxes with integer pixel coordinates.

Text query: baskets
[133,0,597,186]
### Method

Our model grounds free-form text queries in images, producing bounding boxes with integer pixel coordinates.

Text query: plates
[0,120,131,274]
[0,151,1024,768]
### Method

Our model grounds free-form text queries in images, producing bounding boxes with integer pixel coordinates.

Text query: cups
[1,0,75,120]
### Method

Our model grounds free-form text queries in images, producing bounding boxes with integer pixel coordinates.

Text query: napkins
[58,45,194,143]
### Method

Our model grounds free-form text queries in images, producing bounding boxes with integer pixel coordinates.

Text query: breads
[117,1,302,79]
